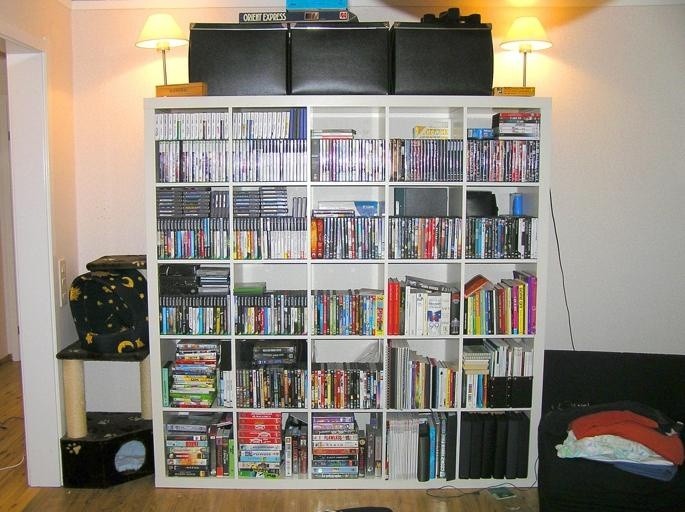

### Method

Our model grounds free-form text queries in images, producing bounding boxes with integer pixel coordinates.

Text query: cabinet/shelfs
[145,96,553,489]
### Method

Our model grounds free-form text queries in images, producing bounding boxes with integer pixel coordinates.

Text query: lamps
[135,13,190,84]
[499,16,552,88]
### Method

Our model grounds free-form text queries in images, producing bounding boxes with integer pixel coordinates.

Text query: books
[459,111,542,478]
[284,0,348,10]
[485,485,517,500]
[84,254,146,271]
[238,9,350,24]
[312,128,387,478]
[492,86,536,97]
[385,127,464,481]
[155,82,209,96]
[155,112,235,478]
[232,107,309,477]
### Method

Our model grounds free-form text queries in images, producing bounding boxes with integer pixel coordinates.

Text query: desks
[56,340,148,440]
